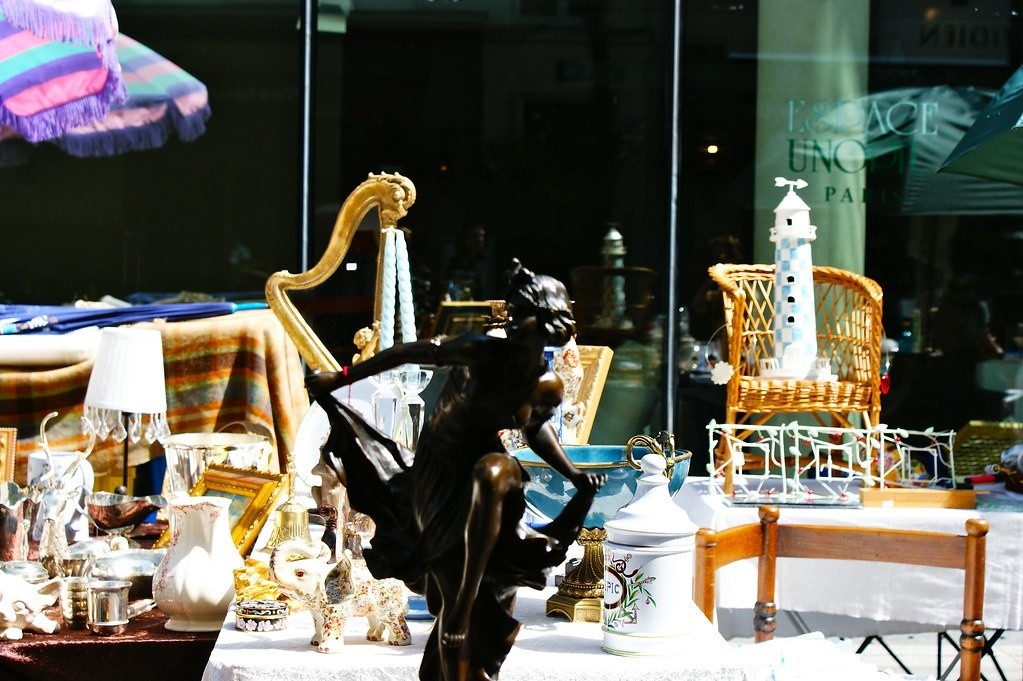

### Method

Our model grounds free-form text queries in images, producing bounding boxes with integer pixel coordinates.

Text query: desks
[0,598,220,681]
[203,483,744,681]
[673,475,1023,681]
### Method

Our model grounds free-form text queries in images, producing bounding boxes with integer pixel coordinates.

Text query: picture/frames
[151,461,294,558]
[576,344,613,445]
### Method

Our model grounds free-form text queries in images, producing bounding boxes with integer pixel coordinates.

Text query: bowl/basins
[506,442,693,530]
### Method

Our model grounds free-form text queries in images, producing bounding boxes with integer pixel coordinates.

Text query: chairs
[707,261,885,495]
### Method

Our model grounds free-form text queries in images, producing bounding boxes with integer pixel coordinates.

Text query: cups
[27,450,96,542]
[87,581,131,637]
[161,429,273,498]
[57,577,100,629]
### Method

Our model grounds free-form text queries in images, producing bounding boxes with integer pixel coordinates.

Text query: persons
[307,278,596,681]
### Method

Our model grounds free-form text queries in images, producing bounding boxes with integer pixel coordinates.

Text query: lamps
[79,327,171,494]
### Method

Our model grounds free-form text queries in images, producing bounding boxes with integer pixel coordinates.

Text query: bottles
[602,455,697,656]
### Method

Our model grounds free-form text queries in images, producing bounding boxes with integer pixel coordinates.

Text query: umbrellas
[0,0,212,157]
